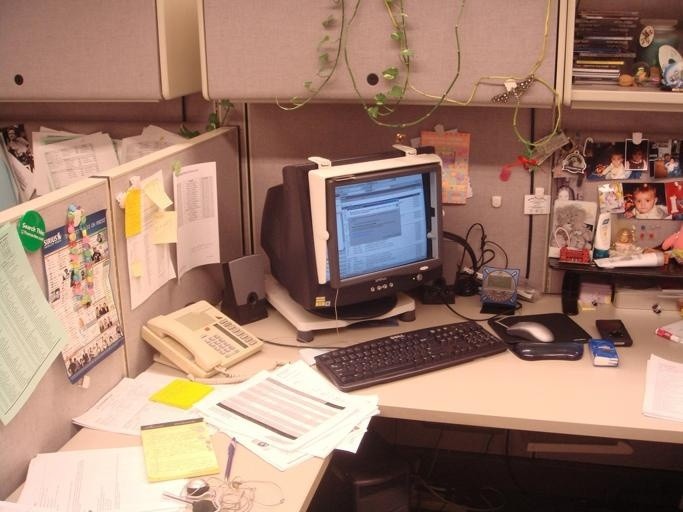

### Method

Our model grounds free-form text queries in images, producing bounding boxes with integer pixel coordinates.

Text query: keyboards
[314,320,508,393]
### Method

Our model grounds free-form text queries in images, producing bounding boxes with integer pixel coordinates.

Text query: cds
[658,45,683,73]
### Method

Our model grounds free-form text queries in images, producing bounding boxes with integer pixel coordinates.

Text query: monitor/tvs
[260,146,442,321]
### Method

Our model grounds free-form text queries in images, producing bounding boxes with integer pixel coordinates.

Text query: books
[140,417,221,484]
[573,7,641,85]
[654,319,683,344]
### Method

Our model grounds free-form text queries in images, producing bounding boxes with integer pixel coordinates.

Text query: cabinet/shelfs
[1,1,200,105]
[198,0,568,109]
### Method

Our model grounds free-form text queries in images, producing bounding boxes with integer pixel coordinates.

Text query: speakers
[422,237,456,305]
[222,254,267,327]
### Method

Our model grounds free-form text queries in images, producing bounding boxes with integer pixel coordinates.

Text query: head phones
[442,229,479,298]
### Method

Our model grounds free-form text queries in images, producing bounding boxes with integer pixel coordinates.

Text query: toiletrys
[589,249,670,270]
[594,210,612,260]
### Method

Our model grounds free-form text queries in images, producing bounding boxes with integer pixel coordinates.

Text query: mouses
[504,321,555,345]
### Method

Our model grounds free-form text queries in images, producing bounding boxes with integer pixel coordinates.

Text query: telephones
[141,300,264,379]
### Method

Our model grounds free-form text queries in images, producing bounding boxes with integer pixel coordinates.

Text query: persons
[624,184,667,220]
[627,148,647,170]
[50,245,122,375]
[601,152,626,180]
[5,126,32,162]
[662,152,680,176]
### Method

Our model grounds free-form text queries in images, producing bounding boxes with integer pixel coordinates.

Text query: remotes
[596,319,637,347]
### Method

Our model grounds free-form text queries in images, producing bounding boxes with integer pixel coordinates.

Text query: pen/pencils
[223,437,236,481]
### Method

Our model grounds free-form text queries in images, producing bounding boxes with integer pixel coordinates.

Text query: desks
[4,293,683,512]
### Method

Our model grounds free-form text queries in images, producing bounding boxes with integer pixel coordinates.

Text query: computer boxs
[346,438,412,512]
